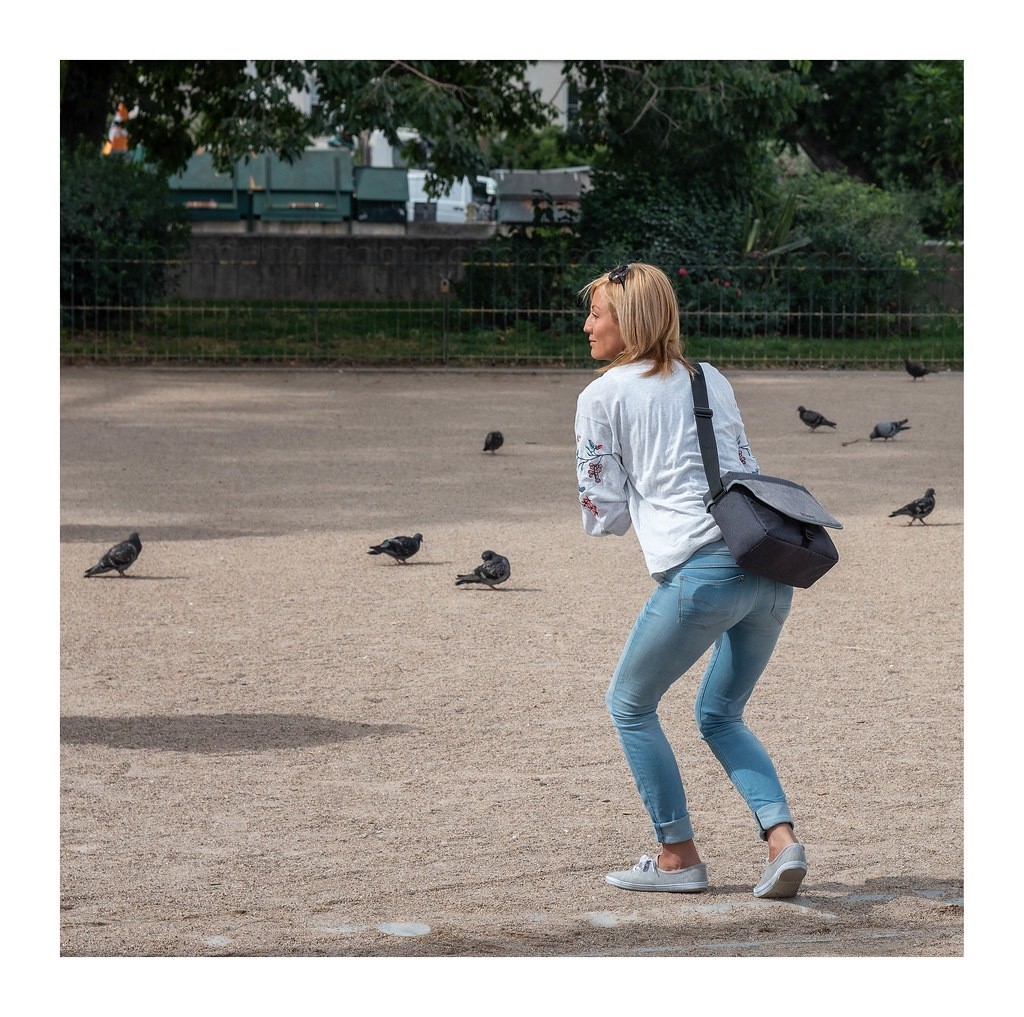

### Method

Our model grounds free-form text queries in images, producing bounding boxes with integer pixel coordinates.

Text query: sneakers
[605,855,708,892]
[753,843,807,898]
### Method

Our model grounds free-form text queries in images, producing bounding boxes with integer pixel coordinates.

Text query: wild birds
[870,419,911,443]
[455,550,511,591]
[888,488,936,526]
[366,533,424,567]
[901,358,938,382]
[483,431,503,455]
[797,406,837,433]
[83,530,142,577]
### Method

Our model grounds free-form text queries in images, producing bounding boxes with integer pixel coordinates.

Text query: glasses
[608,266,629,292]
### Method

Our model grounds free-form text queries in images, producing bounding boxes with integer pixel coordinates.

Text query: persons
[573,262,807,897]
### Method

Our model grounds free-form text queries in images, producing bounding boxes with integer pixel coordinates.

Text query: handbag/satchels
[702,468,844,590]
